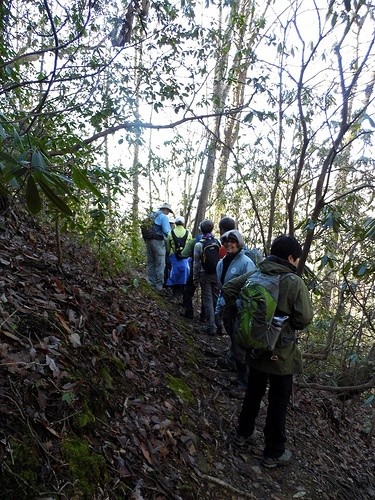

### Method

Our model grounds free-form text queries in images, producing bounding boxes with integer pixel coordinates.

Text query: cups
[273,316,288,329]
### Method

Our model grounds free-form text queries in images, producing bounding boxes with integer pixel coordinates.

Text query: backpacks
[233,272,293,359]
[199,238,219,275]
[171,229,188,250]
[244,248,264,270]
[140,213,163,242]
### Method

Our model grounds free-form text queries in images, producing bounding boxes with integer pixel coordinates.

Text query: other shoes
[229,387,246,398]
[218,324,227,334]
[217,357,227,369]
[200,314,205,321]
[180,312,193,318]
[206,326,217,335]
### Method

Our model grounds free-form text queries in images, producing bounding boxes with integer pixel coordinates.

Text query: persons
[222,235,313,469]
[141,205,263,358]
[214,229,256,390]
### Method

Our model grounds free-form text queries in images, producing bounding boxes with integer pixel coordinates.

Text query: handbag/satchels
[166,253,189,285]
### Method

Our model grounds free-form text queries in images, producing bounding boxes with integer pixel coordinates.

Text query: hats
[157,203,173,213]
[168,217,175,223]
[201,221,214,232]
[220,218,235,229]
[223,234,237,242]
[175,216,185,223]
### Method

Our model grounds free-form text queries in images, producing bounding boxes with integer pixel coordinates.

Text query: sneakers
[235,427,257,445]
[261,449,292,468]
